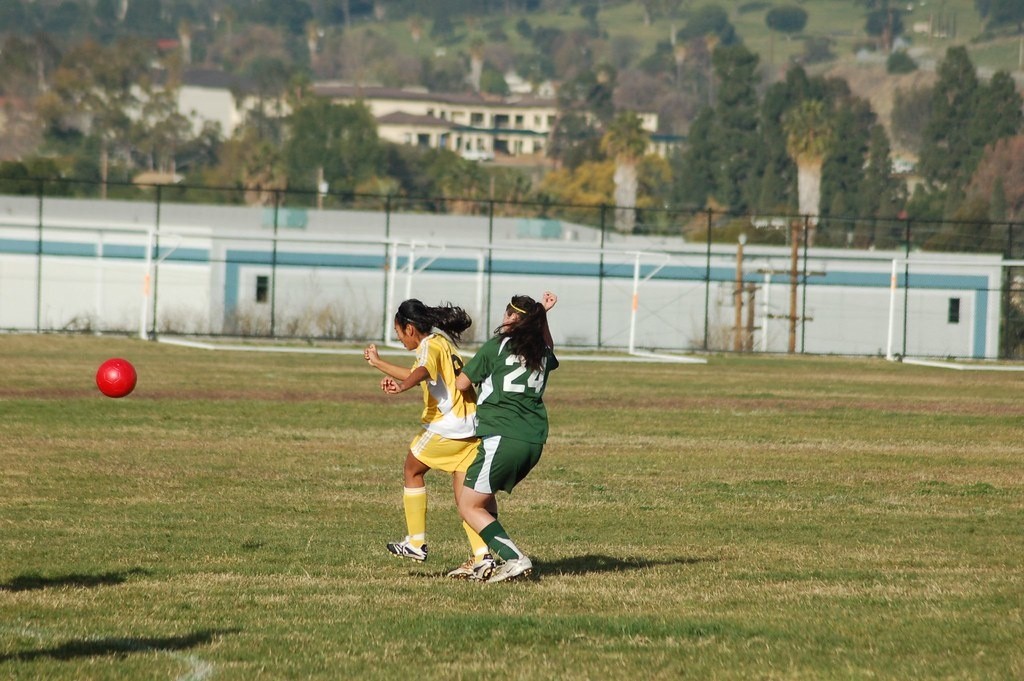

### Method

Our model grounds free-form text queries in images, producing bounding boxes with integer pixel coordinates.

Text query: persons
[364,298,496,581]
[447,291,560,583]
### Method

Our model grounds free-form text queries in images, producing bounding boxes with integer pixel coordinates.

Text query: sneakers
[486,556,533,584]
[466,554,496,582]
[387,541,427,563]
[448,560,474,579]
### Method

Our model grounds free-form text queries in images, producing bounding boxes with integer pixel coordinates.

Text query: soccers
[96,357,137,399]
